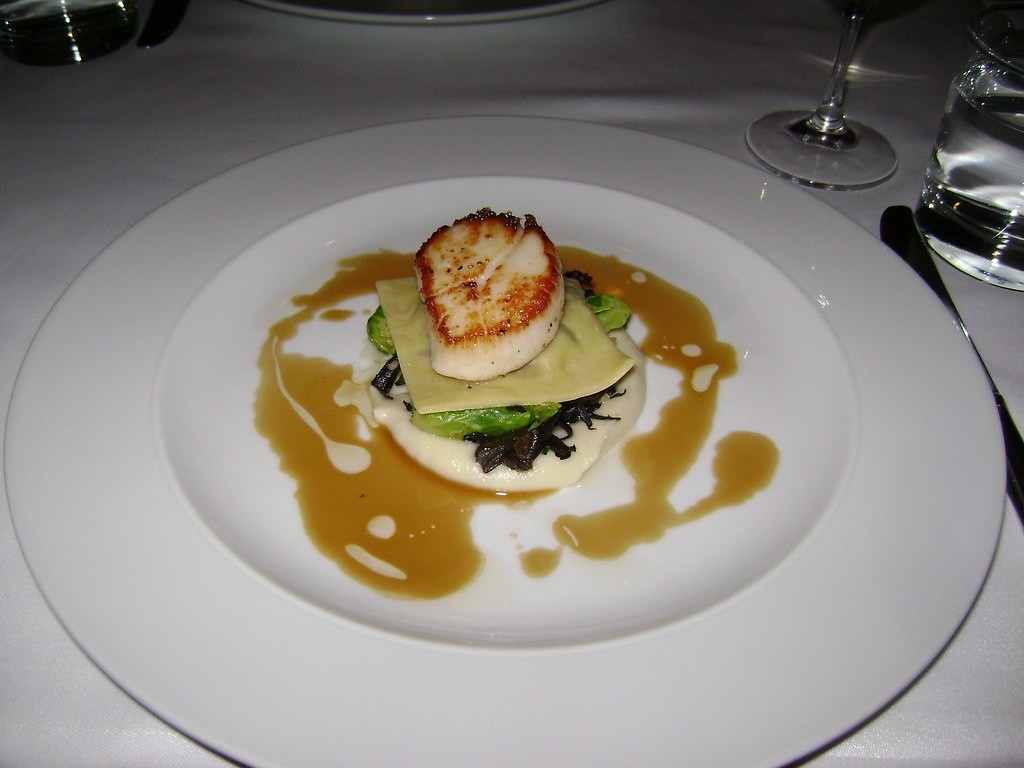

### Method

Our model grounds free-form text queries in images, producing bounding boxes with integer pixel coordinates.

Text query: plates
[4,116,1007,768]
[246,0,608,26]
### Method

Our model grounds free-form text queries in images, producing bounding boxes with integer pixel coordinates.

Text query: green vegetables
[367,267,633,470]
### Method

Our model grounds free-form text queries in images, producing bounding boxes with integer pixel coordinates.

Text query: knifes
[136,0,191,48]
[879,205,1024,530]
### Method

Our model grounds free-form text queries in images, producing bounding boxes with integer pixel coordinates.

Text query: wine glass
[743,0,899,191]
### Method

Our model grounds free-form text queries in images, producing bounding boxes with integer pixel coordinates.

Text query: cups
[1,0,138,67]
[915,6,1024,292]
